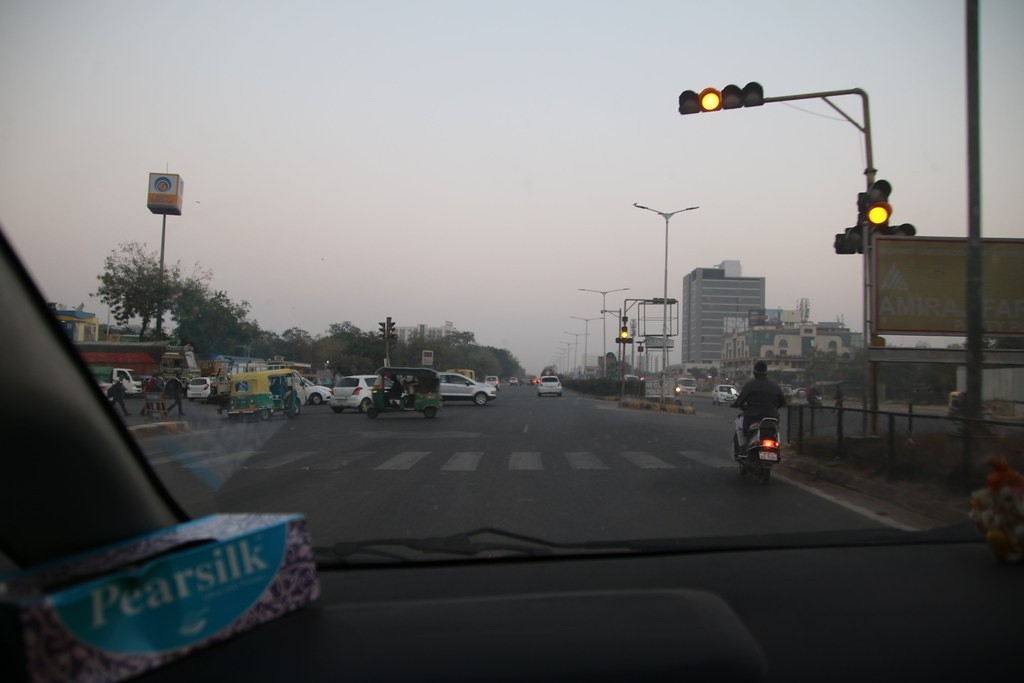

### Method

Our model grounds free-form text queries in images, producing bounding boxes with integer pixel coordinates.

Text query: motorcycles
[366,366,441,419]
[225,369,301,421]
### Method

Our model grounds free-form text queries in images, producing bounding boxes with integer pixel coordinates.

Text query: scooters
[809,392,822,408]
[730,388,782,485]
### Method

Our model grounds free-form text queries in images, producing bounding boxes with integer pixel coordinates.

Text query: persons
[385,375,403,407]
[140,370,169,416]
[280,375,288,394]
[315,377,321,386]
[111,374,132,417]
[399,375,424,407]
[806,386,822,405]
[272,377,281,395]
[830,384,844,414]
[730,359,786,462]
[166,372,185,415]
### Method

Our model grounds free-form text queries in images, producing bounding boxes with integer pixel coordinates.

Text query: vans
[673,377,698,398]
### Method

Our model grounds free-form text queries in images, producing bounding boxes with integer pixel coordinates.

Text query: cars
[509,376,540,385]
[722,375,807,403]
[97,368,332,416]
[536,375,562,397]
[330,374,408,414]
[711,384,739,407]
[433,368,502,407]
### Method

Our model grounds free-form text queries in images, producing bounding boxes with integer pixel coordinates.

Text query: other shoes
[125,413,131,416]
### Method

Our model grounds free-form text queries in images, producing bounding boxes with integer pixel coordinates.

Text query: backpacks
[108,382,121,397]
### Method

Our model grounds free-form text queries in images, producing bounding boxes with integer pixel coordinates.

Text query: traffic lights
[679,81,764,115]
[389,321,396,335]
[866,180,916,237]
[621,326,628,339]
[379,322,386,338]
[616,338,633,343]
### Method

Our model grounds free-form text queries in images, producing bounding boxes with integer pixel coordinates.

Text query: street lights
[560,342,580,375]
[571,317,604,378]
[563,331,590,375]
[577,287,631,376]
[636,205,700,412]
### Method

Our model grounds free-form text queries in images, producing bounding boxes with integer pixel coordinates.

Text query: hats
[753,361,767,377]
